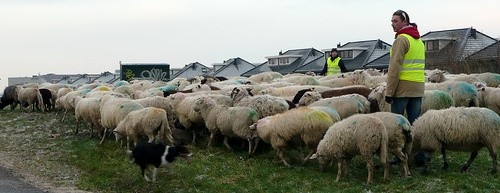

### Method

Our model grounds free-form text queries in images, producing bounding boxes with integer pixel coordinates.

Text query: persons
[385,10,426,167]
[319,48,349,76]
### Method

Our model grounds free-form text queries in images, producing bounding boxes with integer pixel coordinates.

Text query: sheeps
[408,69,499,173]
[0,75,233,150]
[193,68,413,185]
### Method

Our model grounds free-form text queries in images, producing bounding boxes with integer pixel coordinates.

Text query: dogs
[125,142,193,181]
